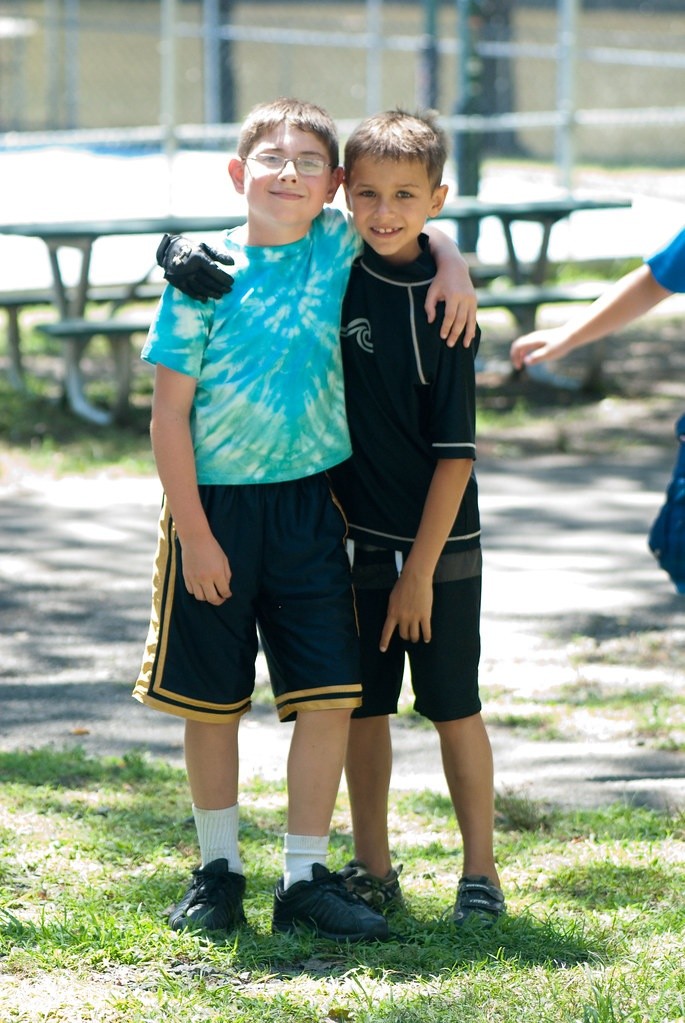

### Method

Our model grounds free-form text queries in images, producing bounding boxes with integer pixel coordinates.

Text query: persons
[133,96,477,938]
[157,112,503,925]
[511,227,685,602]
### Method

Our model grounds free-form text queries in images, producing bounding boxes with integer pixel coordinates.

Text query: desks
[0,199,635,414]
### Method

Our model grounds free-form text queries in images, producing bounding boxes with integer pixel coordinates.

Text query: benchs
[0,278,611,427]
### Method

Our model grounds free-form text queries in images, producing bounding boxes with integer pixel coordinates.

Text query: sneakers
[333,858,404,909]
[167,859,247,933]
[454,875,504,934]
[272,862,388,942]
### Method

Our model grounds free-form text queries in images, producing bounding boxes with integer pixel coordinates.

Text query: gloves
[155,233,235,302]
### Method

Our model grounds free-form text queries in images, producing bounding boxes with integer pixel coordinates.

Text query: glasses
[243,153,332,177]
